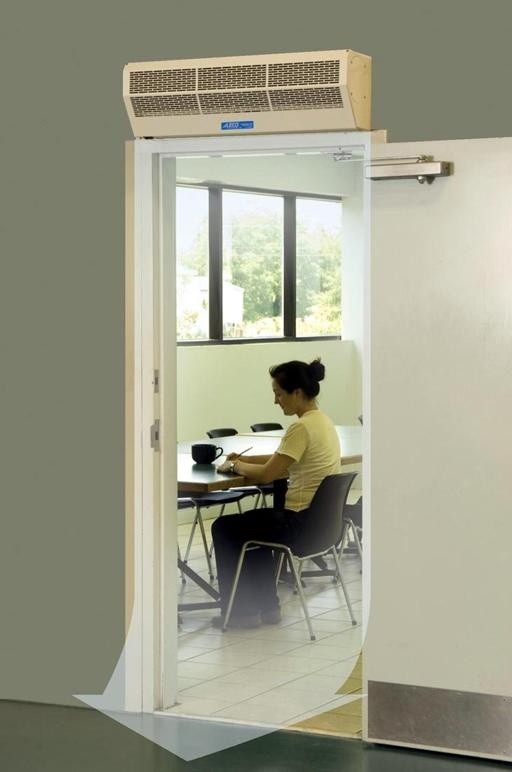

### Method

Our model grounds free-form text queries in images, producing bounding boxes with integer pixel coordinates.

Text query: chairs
[187,421,365,637]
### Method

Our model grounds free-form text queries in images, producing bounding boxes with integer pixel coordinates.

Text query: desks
[180,422,363,635]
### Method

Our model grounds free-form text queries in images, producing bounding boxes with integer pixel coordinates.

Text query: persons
[211,359,341,629]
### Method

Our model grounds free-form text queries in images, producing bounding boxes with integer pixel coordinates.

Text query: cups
[191,444,223,465]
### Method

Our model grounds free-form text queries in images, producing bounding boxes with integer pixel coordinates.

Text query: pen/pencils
[237,446,254,458]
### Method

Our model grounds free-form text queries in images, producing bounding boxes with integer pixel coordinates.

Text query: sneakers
[212,610,280,630]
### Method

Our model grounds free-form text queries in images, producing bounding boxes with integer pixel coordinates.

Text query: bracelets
[230,460,240,472]
